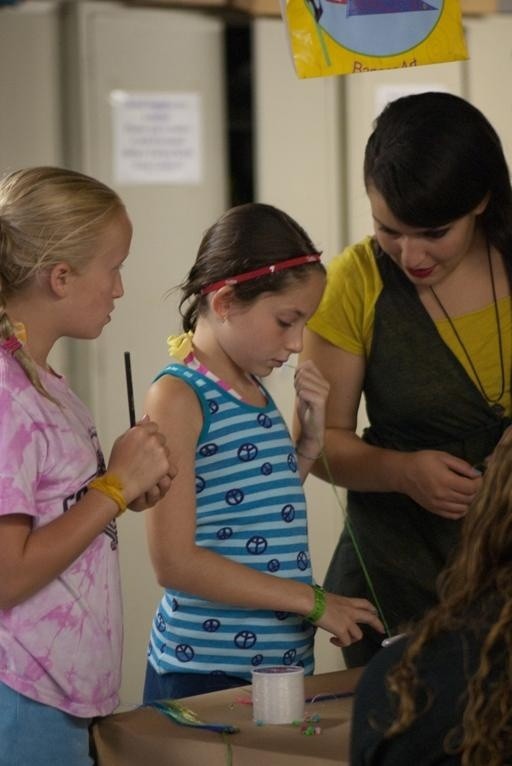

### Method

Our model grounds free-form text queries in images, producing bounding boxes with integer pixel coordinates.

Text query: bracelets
[295,582,328,626]
[87,473,127,518]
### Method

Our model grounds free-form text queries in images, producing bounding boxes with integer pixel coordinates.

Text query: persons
[143,201,388,706]
[353,424,511,766]
[1,160,177,765]
[289,91,512,670]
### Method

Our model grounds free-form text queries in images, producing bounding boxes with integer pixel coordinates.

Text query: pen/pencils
[124,352,135,427]
[306,692,353,702]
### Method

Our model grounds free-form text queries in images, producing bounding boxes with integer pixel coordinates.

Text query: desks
[91,664,364,765]
